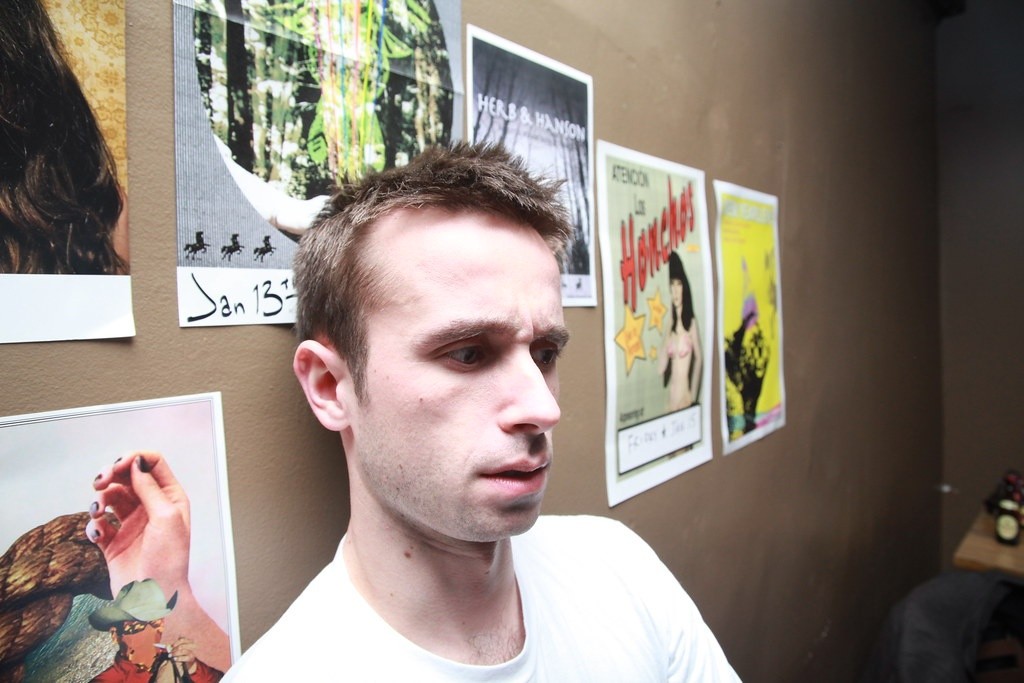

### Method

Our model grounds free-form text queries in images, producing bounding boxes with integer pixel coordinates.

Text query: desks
[957,510,1024,586]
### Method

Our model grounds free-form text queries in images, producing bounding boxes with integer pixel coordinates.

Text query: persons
[218,143,740,683]
[86,450,232,673]
[660,250,703,411]
